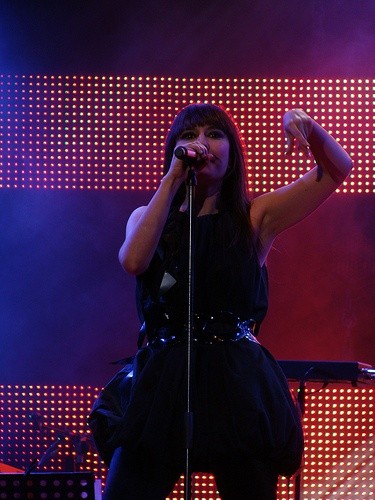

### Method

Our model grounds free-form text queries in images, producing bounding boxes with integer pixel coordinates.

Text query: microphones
[175,146,202,161]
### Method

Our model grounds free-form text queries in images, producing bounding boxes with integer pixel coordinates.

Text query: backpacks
[89,364,134,467]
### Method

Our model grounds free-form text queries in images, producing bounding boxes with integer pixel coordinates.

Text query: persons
[84,101,356,500]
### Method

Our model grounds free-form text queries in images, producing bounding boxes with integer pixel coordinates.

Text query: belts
[141,311,260,345]
